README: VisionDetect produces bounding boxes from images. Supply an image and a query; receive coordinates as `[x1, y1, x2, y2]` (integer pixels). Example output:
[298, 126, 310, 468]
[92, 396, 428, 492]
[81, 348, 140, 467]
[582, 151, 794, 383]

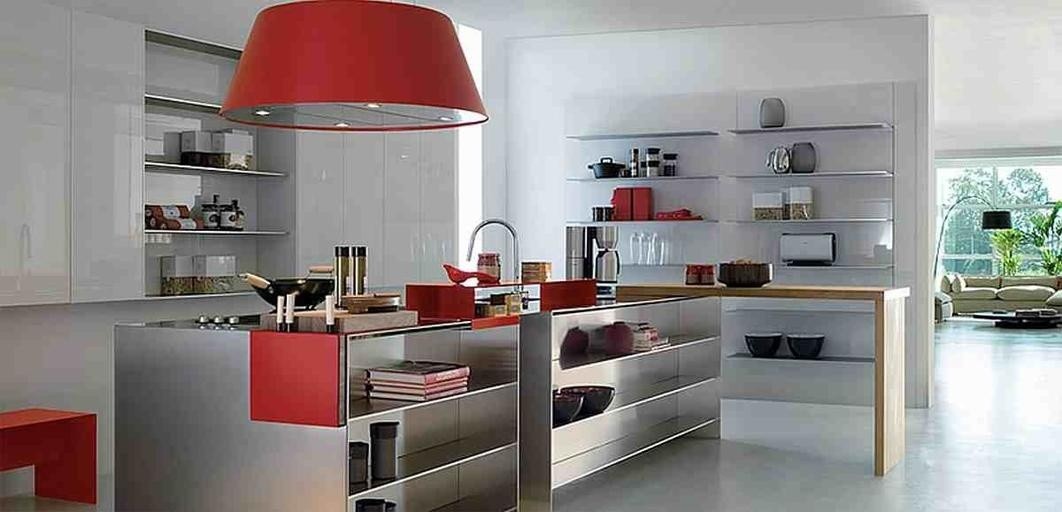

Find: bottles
[370, 422, 400, 478]
[348, 442, 369, 481]
[354, 498, 397, 512]
[630, 148, 678, 177]
[630, 232, 671, 265]
[333, 245, 366, 309]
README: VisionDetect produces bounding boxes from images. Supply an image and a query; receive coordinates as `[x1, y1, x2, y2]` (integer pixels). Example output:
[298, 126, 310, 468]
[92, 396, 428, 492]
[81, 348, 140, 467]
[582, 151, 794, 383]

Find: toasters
[778, 231, 838, 267]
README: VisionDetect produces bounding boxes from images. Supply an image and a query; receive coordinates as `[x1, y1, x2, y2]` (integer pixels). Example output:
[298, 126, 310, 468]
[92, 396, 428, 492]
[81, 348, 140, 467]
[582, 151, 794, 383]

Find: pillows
[952, 274, 966, 293]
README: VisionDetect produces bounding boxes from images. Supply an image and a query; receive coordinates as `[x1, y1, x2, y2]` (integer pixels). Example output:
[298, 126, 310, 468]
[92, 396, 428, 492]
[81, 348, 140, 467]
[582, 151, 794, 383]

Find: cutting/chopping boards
[260, 310, 420, 333]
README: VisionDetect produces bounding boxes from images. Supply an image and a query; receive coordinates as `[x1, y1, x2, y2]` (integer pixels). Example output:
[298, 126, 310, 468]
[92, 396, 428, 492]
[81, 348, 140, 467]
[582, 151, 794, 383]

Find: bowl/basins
[787, 334, 825, 360]
[745, 333, 782, 357]
[561, 386, 615, 413]
[553, 391, 584, 425]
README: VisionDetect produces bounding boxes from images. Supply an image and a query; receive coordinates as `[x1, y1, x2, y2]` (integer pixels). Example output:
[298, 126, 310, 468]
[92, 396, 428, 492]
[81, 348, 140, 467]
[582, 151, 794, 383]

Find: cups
[592, 207, 603, 221]
[605, 207, 616, 220]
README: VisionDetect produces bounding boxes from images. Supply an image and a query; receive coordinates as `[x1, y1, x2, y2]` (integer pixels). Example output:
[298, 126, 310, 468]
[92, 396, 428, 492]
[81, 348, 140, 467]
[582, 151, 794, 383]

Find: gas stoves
[196, 303, 319, 325]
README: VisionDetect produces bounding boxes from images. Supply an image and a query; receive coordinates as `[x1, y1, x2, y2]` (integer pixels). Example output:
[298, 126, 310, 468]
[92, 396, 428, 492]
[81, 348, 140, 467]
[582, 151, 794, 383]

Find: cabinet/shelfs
[258, 126, 459, 298]
[520, 294, 721, 512]
[146, 28, 298, 299]
[0, 0, 146, 308]
[344, 318, 518, 511]
[568, 121, 892, 407]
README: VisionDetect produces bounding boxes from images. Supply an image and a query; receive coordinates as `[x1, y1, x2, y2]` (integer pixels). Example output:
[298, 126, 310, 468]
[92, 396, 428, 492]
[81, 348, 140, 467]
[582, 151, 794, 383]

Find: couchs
[934, 291, 953, 321]
[941, 270, 1062, 322]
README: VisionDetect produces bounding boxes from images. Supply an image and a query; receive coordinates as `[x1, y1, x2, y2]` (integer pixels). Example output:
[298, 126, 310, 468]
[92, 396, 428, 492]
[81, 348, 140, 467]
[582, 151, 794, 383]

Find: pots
[238, 266, 336, 310]
[588, 157, 626, 178]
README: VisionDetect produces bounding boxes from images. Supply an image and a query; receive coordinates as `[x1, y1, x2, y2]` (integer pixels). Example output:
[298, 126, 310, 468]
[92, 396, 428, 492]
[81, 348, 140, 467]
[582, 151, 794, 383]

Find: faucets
[466, 217, 520, 294]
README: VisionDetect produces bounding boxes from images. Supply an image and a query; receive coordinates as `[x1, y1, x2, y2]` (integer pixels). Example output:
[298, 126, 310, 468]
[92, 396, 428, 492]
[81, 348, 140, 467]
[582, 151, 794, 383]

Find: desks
[617, 285, 912, 474]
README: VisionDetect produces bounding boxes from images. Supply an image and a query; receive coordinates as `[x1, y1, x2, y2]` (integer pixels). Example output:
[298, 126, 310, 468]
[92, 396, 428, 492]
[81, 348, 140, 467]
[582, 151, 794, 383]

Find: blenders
[566, 226, 620, 299]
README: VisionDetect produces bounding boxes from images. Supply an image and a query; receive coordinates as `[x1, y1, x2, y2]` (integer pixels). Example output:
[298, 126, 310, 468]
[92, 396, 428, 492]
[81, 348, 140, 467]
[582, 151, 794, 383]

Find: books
[614, 320, 670, 351]
[369, 360, 470, 402]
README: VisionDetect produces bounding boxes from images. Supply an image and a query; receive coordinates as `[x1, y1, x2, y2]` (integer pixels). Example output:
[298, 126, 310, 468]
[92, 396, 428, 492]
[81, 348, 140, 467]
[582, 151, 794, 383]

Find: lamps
[221, 1, 486, 128]
[934, 195, 1012, 276]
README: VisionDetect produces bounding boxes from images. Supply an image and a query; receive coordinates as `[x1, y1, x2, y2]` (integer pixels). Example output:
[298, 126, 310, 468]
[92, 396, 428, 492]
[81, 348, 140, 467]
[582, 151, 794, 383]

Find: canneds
[200, 203, 244, 231]
[522, 262, 552, 283]
[684, 264, 715, 286]
[477, 251, 501, 283]
